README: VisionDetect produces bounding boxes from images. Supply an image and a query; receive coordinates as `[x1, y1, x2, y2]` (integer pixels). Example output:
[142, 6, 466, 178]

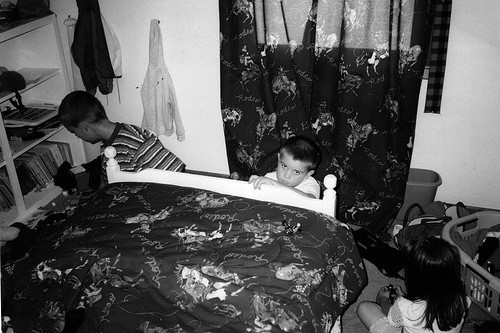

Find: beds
[1, 145, 368, 333]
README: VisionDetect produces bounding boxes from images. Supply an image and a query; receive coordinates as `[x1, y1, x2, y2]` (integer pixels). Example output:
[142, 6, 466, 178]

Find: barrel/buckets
[397, 168, 442, 220]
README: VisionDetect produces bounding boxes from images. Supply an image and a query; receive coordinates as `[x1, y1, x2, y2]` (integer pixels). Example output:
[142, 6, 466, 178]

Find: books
[0, 141, 74, 211]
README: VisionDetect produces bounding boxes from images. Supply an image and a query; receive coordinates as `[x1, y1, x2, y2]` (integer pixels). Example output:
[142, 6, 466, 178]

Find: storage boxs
[440, 210, 500, 321]
[395, 168, 443, 223]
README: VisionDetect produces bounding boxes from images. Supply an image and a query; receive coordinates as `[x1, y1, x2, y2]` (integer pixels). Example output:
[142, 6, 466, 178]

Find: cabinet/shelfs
[0, 12, 88, 228]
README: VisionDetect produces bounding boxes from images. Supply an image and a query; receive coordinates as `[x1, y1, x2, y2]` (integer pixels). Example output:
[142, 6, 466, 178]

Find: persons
[58, 90, 187, 188]
[247, 137, 320, 200]
[357, 235, 471, 333]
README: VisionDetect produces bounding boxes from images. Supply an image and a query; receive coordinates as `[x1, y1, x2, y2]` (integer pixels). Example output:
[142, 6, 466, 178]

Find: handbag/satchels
[393, 203, 453, 252]
